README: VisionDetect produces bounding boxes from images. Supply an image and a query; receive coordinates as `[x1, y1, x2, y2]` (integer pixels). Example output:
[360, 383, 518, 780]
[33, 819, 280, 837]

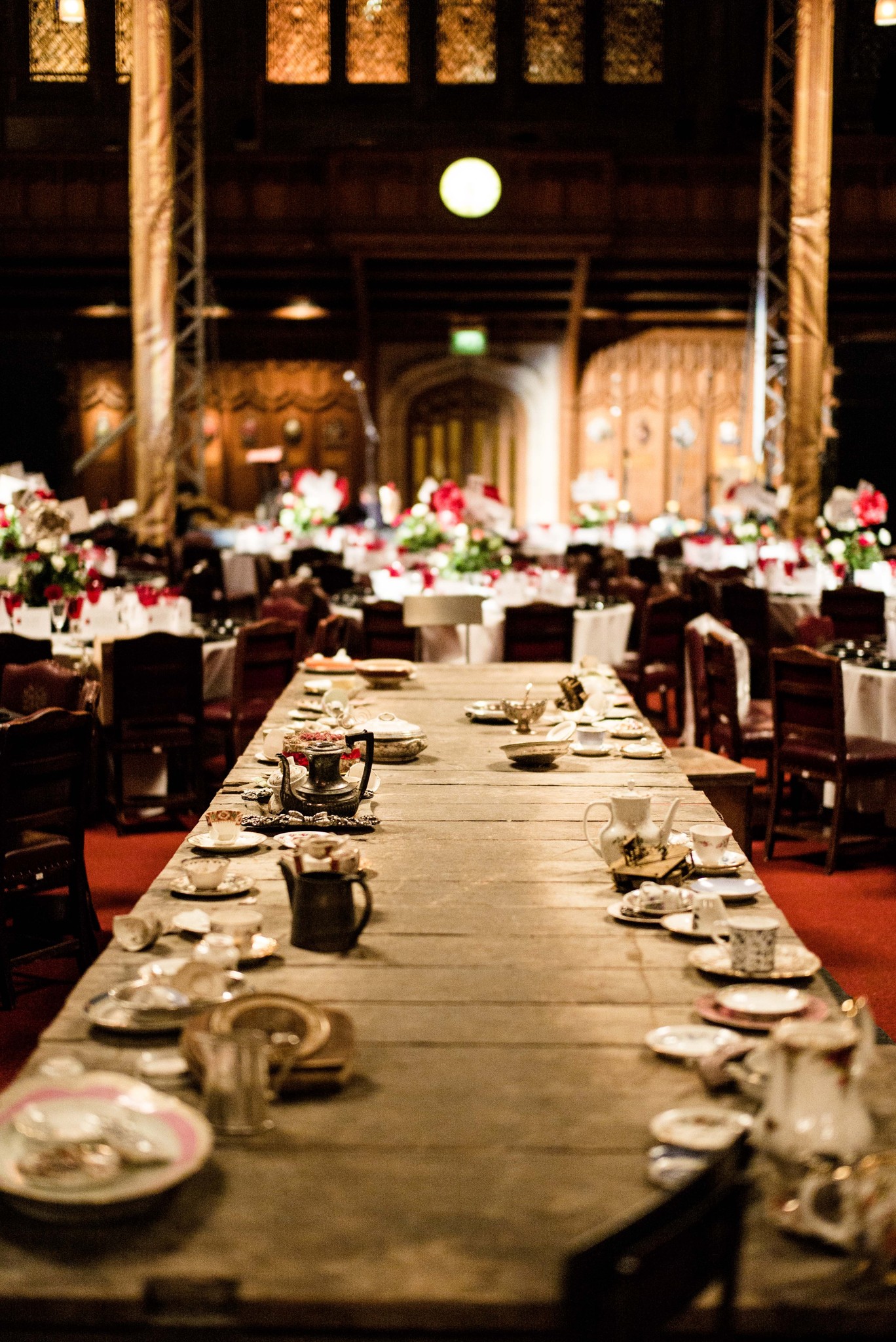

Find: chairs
[0, 507, 894, 998]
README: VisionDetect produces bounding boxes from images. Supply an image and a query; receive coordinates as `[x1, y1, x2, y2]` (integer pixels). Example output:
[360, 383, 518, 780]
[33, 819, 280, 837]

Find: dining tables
[1, 650, 896, 1342]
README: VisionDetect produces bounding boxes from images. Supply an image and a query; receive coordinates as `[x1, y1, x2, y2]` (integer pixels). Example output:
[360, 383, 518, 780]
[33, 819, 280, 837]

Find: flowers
[0, 456, 894, 647]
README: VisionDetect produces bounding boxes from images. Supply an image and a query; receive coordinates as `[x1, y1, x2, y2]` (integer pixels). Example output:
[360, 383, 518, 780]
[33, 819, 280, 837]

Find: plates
[0, 635, 896, 1256]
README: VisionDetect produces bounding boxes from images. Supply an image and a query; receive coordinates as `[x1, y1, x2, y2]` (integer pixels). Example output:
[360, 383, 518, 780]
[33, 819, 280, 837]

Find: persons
[167, 467, 492, 613]
